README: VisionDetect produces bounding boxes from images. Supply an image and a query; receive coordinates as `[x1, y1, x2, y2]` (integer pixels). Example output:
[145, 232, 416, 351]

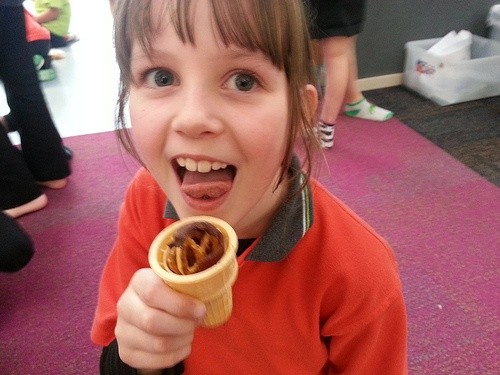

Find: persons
[304, 0, 393, 148]
[0, 0, 72, 217]
[32, 0, 71, 47]
[25, 10, 64, 80]
[88, 0, 409, 375]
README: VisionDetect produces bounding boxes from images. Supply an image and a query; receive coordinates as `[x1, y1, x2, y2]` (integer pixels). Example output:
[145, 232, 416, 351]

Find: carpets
[0, 101, 500, 375]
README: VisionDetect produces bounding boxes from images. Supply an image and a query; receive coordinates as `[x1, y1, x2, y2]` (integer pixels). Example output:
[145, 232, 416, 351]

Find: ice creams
[147, 215, 239, 328]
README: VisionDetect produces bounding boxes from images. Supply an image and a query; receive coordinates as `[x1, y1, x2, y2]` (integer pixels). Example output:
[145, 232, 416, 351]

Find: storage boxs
[403, 36, 500, 106]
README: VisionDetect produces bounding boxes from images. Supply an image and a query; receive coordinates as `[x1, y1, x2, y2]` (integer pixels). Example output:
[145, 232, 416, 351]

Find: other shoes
[38, 178, 67, 188]
[0, 193, 48, 218]
[49, 32, 76, 49]
